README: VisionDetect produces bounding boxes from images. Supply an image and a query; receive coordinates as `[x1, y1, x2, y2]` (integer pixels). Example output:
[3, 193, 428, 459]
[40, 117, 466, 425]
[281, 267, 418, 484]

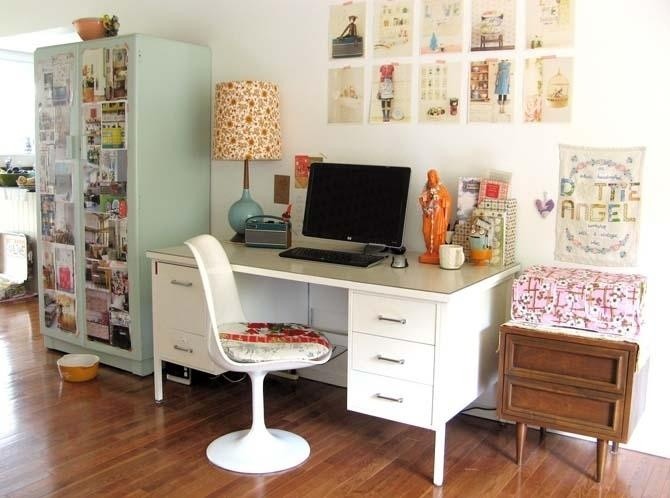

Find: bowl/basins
[0, 173, 28, 187]
[57, 353, 100, 382]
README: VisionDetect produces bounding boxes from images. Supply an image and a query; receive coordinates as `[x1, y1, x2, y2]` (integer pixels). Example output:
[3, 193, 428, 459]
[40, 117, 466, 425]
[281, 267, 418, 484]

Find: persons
[420, 170, 451, 256]
[337, 16, 357, 39]
[377, 63, 394, 121]
[495, 60, 512, 114]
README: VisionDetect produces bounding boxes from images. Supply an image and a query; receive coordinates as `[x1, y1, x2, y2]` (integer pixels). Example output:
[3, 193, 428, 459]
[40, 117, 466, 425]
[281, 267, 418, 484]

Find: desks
[146, 240, 522, 486]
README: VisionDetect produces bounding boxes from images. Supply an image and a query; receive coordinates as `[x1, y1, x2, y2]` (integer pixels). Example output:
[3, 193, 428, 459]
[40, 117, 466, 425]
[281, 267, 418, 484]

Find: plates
[19, 185, 36, 191]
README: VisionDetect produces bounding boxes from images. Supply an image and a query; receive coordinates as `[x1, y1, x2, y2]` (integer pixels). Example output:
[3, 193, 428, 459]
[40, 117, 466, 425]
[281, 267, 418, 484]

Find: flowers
[104, 14, 119, 34]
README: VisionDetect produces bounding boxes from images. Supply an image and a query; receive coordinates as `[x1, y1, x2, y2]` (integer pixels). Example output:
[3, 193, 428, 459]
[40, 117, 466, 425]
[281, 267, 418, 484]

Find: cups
[83, 87, 94, 101]
[439, 244, 465, 269]
[102, 128, 122, 149]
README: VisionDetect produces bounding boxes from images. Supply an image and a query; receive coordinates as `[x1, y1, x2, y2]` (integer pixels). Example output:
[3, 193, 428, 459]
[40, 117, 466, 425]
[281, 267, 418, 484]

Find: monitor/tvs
[302, 162, 411, 255]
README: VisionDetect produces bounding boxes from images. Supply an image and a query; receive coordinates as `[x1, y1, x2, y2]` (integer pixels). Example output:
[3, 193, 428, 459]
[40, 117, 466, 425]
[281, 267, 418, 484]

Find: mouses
[390, 255, 408, 268]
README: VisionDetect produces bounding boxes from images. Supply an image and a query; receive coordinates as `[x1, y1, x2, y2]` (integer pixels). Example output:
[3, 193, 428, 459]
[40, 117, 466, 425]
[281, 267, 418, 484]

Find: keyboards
[279, 247, 384, 269]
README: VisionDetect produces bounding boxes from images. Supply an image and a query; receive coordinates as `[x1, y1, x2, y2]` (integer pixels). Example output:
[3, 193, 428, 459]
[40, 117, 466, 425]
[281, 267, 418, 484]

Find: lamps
[210, 81, 279, 242]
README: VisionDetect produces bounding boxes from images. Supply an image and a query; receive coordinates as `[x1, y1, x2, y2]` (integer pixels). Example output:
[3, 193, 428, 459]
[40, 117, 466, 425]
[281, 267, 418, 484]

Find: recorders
[245, 215, 292, 249]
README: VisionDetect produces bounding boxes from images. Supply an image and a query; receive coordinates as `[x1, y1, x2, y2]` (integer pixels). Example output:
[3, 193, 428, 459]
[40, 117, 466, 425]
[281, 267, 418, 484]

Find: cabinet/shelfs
[33, 33, 213, 376]
[496, 322, 638, 482]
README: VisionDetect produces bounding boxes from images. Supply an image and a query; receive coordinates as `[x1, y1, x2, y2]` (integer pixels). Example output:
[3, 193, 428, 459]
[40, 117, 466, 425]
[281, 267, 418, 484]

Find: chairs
[185, 235, 331, 473]
